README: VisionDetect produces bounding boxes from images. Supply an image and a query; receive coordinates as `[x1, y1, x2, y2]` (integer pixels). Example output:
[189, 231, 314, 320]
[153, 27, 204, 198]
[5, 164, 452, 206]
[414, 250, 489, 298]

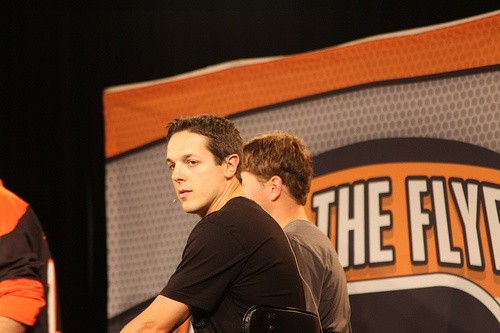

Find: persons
[241, 134, 352, 333]
[119, 114, 306, 333]
[0, 180, 60, 333]
